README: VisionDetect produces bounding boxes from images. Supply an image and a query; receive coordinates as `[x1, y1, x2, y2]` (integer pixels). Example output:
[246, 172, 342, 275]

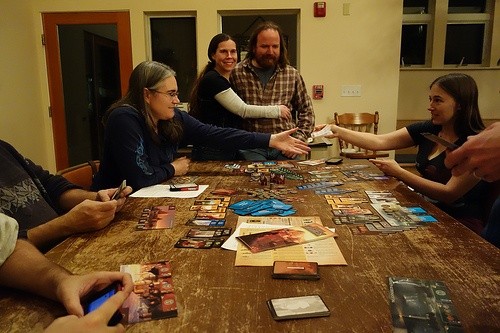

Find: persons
[0, 213, 134, 333]
[92, 61, 312, 197]
[314, 73, 487, 236]
[443, 122, 500, 183]
[0, 139, 133, 254]
[229, 23, 316, 160]
[187, 33, 291, 161]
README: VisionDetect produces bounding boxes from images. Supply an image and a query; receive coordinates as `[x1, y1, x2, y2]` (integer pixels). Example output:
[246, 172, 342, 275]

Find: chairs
[334, 110, 389, 163]
[57, 161, 100, 189]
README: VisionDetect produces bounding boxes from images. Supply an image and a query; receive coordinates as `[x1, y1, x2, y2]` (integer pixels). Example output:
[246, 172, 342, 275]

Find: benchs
[396, 119, 500, 177]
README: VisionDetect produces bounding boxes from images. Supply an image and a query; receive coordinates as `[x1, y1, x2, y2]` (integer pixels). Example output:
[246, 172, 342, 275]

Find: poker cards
[176, 158, 438, 250]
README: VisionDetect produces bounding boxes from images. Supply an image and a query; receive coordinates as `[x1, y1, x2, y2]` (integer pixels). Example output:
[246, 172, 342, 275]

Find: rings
[473, 172, 483, 180]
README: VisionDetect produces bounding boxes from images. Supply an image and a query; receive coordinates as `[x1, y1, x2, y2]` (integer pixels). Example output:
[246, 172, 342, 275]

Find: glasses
[148, 89, 180, 99]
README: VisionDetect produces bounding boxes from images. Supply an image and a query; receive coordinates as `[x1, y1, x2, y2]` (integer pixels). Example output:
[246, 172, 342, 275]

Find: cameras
[80, 281, 127, 326]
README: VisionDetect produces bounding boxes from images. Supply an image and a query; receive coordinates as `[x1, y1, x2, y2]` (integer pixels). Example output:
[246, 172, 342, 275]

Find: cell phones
[325, 157, 343, 165]
[170, 183, 199, 191]
[109, 180, 126, 200]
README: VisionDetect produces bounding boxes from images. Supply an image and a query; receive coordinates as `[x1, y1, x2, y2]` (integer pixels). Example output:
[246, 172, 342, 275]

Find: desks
[0, 159, 500, 333]
